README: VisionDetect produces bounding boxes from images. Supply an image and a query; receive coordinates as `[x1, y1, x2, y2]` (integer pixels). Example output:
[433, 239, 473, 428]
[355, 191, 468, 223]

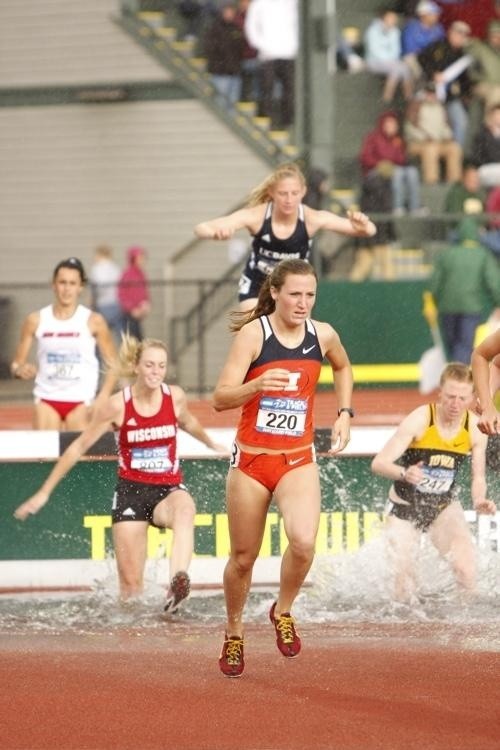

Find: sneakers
[219, 631, 244, 678]
[269, 602, 300, 658]
[164, 572, 189, 614]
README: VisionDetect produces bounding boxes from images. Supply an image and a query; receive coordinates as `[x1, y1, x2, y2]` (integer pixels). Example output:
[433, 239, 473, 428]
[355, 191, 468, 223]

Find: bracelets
[336, 406, 356, 418]
[15, 362, 28, 378]
[401, 468, 410, 483]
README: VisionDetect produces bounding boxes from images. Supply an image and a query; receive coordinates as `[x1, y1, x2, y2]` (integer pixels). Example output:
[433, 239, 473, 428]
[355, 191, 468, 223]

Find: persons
[211, 257, 357, 680]
[434, 215, 499, 366]
[368, 356, 498, 625]
[194, 0, 298, 125]
[471, 331, 500, 436]
[9, 257, 124, 430]
[85, 242, 121, 364]
[12, 339, 230, 623]
[116, 245, 151, 367]
[195, 162, 378, 314]
[338, 1, 499, 253]
